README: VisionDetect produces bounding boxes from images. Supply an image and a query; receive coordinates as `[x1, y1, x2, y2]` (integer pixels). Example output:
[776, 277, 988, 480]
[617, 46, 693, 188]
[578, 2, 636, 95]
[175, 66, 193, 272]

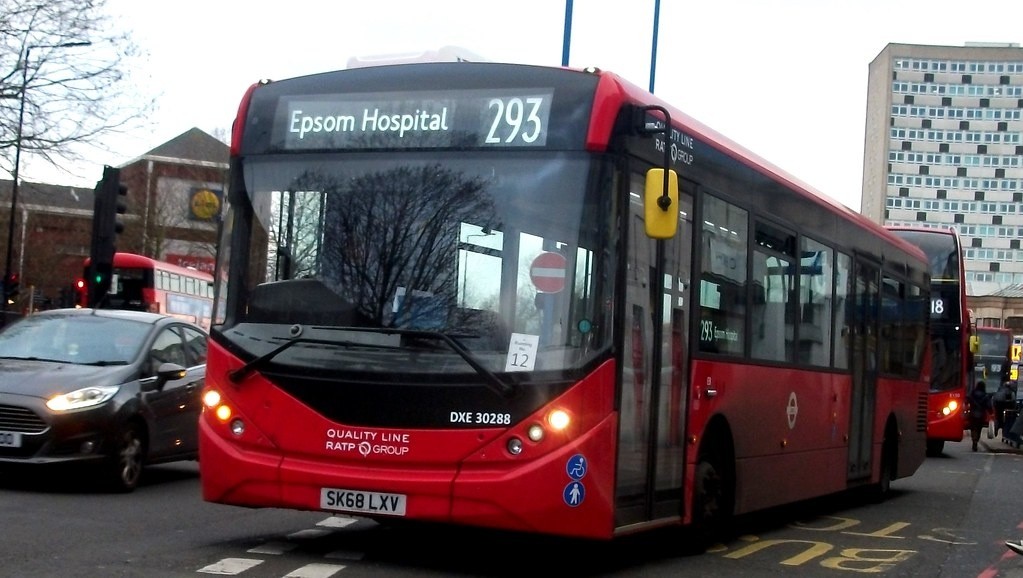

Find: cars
[0, 308, 222, 494]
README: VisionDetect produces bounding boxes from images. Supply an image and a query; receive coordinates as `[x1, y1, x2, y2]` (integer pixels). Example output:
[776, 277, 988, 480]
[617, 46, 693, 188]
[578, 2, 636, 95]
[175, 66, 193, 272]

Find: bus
[198, 56, 932, 550]
[80, 254, 225, 340]
[872, 223, 978, 456]
[970, 325, 1020, 429]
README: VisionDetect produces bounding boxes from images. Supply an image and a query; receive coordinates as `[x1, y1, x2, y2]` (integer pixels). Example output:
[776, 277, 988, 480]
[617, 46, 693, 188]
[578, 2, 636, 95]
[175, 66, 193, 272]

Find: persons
[966, 381, 993, 452]
[992, 380, 1016, 437]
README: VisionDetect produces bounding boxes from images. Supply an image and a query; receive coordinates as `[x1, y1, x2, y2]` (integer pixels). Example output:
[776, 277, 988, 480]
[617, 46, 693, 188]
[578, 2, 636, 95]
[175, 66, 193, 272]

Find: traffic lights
[97, 163, 129, 240]
[4, 273, 20, 299]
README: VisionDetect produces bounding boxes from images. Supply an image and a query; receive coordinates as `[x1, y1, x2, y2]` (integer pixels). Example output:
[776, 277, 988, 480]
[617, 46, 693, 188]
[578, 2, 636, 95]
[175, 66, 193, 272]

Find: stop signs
[530, 251, 569, 294]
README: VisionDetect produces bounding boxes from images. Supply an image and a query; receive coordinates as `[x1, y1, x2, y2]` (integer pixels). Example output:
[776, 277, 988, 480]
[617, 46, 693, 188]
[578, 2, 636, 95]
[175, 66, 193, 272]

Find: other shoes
[972, 447, 977, 452]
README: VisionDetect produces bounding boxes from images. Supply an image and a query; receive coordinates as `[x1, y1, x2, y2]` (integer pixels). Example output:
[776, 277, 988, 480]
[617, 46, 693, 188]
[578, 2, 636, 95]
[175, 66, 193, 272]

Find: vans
[1013, 335, 1023, 365]
